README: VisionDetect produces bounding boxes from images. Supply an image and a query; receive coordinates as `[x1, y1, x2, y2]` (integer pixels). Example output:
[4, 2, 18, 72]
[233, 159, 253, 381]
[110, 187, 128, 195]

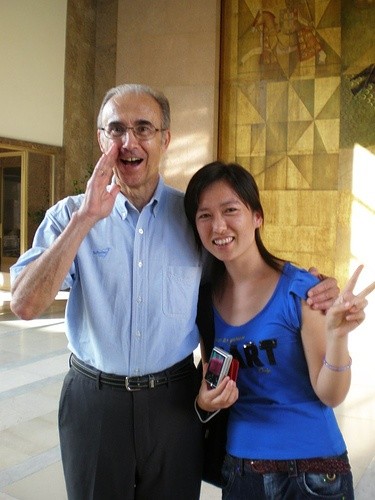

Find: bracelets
[324, 359, 353, 371]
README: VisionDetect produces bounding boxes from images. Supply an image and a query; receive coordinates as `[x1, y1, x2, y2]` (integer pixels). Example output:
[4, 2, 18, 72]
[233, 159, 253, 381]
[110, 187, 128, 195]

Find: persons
[8, 84, 340, 500]
[184, 161, 375, 500]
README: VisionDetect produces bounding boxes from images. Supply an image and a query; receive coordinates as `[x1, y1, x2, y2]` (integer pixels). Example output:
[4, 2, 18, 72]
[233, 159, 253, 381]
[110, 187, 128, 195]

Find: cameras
[204, 346, 233, 389]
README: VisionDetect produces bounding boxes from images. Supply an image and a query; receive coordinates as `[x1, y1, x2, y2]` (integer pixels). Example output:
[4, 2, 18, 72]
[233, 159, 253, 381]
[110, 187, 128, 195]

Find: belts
[233, 458, 352, 476]
[71, 355, 196, 391]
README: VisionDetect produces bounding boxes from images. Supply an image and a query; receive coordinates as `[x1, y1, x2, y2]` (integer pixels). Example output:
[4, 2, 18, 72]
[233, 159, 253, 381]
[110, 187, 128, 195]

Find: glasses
[98, 123, 167, 141]
